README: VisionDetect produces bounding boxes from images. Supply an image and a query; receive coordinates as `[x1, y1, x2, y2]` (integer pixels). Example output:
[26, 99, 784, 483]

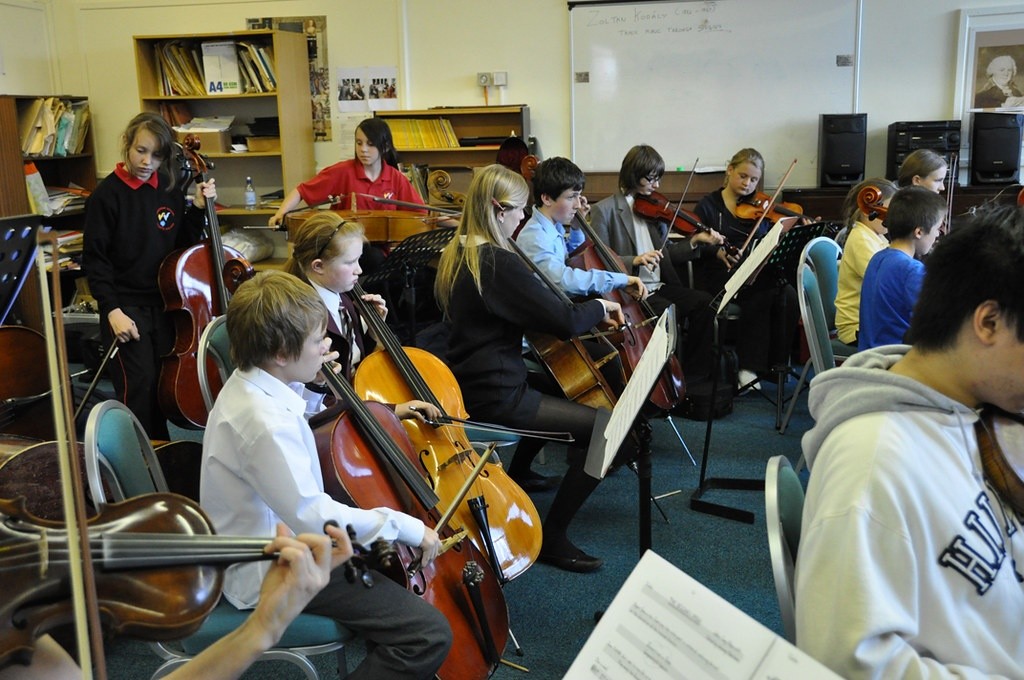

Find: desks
[782, 186, 1023, 231]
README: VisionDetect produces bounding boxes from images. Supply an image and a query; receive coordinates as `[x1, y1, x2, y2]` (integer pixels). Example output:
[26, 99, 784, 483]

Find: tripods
[734, 220, 830, 427]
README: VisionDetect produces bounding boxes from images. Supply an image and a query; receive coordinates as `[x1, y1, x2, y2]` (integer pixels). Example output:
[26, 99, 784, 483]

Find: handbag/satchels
[676, 371, 738, 421]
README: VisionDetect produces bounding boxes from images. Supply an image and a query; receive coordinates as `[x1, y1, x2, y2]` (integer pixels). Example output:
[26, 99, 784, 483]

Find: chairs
[463, 428, 545, 472]
[765, 455, 807, 646]
[197, 315, 235, 415]
[83, 399, 354, 679]
[688, 257, 793, 382]
[779, 227, 858, 435]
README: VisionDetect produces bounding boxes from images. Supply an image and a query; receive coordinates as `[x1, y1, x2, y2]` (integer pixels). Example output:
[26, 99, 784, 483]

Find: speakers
[971, 113, 1023, 187]
[818, 113, 867, 188]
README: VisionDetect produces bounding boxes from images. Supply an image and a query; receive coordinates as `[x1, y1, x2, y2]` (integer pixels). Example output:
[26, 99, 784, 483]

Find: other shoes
[508, 470, 562, 493]
[737, 368, 761, 390]
[537, 537, 604, 571]
[737, 383, 750, 397]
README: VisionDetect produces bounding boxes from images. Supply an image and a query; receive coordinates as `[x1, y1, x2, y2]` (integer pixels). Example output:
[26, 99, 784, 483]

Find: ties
[339, 301, 353, 338]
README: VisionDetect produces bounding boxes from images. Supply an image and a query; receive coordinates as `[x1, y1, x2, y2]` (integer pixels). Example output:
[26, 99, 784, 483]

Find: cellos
[242, 207, 463, 244]
[517, 154, 698, 471]
[856, 185, 952, 260]
[424, 170, 673, 528]
[342, 277, 543, 588]
[154, 132, 259, 432]
[220, 256, 511, 680]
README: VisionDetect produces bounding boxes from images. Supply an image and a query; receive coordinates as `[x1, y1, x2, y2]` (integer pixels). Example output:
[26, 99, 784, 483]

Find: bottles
[244, 177, 256, 210]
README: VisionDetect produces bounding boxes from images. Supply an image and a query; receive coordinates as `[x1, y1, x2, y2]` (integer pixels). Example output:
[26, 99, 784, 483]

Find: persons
[795, 215, 1024, 680]
[857, 184, 949, 353]
[898, 149, 948, 196]
[833, 177, 903, 347]
[0, 111, 823, 680]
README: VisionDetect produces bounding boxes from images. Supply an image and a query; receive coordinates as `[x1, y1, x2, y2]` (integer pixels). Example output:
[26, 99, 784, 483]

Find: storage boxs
[176, 131, 233, 153]
[76, 276, 92, 302]
[201, 41, 245, 95]
[245, 136, 280, 152]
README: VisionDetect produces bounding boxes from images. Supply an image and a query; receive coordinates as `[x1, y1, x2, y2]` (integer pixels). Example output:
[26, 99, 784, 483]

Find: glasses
[644, 175, 659, 184]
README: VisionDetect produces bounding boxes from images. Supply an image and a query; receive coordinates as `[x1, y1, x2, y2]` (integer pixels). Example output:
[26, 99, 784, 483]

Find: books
[153, 39, 277, 96]
[380, 117, 460, 148]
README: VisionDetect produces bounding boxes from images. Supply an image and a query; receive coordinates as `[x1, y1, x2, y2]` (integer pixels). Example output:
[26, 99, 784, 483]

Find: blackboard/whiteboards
[568, 0, 862, 190]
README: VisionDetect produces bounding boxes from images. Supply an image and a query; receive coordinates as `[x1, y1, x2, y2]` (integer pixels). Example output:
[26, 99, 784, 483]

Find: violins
[974, 402, 1024, 526]
[632, 190, 742, 257]
[734, 190, 839, 235]
[0, 488, 401, 673]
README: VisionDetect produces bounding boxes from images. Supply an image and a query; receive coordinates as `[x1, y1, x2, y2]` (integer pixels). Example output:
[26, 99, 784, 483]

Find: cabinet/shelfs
[0, 94, 99, 336]
[132, 29, 316, 271]
[374, 105, 534, 172]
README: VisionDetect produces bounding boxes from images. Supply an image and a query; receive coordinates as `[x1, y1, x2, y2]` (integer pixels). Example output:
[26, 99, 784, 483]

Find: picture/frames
[953, 4, 1024, 167]
